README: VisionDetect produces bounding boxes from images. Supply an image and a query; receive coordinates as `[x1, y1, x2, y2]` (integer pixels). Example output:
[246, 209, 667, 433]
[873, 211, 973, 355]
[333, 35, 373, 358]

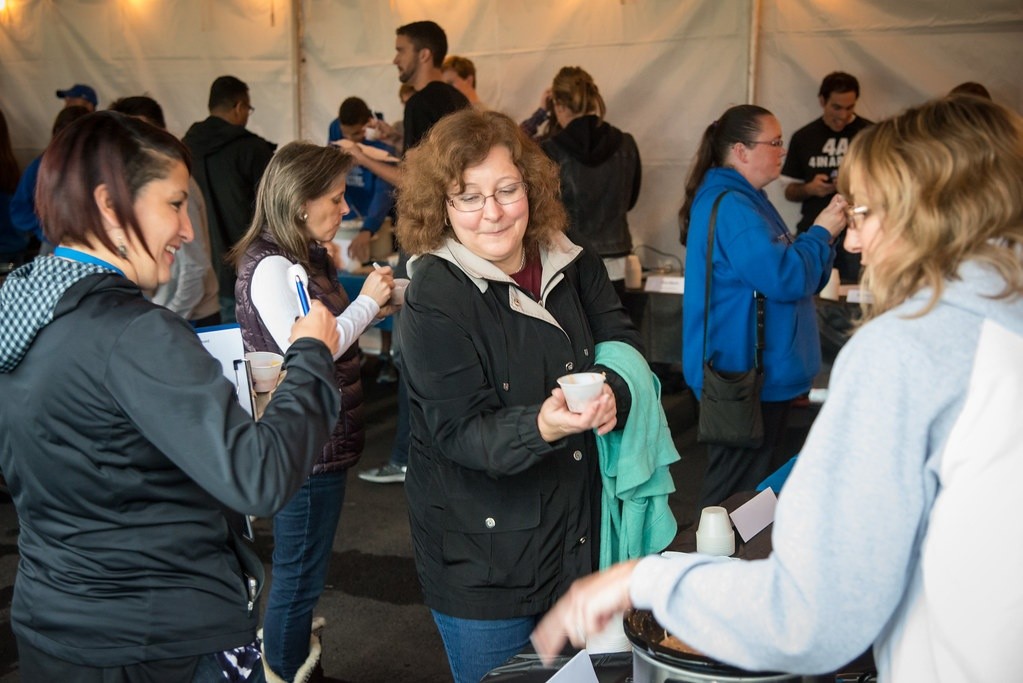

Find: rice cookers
[332, 216, 396, 273]
[622, 606, 837, 682]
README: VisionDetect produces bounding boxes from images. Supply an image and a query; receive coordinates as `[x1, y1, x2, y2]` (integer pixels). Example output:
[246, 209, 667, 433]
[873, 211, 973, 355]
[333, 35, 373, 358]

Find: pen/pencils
[295, 275, 310, 316]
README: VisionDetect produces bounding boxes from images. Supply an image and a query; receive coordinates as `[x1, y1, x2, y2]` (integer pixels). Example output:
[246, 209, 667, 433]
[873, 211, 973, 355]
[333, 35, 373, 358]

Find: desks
[332, 261, 398, 331]
[624, 270, 852, 378]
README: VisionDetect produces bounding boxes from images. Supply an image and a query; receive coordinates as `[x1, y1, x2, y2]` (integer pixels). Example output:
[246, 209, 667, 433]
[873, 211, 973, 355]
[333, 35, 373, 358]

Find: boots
[257, 617, 336, 682]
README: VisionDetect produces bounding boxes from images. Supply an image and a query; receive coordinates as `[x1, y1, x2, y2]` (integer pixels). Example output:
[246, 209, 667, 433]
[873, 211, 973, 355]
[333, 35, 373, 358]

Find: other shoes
[357, 460, 409, 484]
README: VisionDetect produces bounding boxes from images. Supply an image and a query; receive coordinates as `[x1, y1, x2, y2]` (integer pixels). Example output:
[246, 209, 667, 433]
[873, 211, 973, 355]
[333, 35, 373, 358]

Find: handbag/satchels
[696, 360, 767, 449]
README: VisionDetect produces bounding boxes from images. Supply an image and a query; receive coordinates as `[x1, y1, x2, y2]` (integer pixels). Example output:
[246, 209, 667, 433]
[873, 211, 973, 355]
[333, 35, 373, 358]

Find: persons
[677, 104, 849, 510]
[327, 21, 480, 487]
[392, 109, 644, 683]
[522, 68, 640, 309]
[536, 98, 1023, 683]
[0, 109, 343, 683]
[778, 72, 878, 285]
[231, 141, 396, 683]
[0, 75, 278, 338]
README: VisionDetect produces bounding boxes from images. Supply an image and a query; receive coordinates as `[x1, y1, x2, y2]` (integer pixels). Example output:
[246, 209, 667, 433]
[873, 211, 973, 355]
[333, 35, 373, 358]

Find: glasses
[444, 179, 527, 215]
[734, 138, 787, 148]
[841, 204, 877, 229]
[233, 99, 256, 115]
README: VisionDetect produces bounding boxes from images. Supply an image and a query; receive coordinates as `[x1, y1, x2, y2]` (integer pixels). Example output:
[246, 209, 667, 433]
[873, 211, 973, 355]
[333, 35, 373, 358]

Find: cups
[389, 278, 410, 304]
[819, 268, 840, 301]
[625, 254, 641, 288]
[365, 127, 381, 141]
[245, 351, 284, 392]
[696, 506, 735, 557]
[556, 371, 606, 413]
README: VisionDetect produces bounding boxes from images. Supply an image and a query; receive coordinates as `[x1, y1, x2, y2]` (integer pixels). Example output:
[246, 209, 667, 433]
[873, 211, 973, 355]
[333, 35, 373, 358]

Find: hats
[55, 84, 98, 104]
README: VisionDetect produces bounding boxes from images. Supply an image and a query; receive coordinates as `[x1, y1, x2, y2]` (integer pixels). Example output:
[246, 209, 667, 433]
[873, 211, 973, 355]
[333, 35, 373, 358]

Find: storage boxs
[336, 216, 393, 257]
